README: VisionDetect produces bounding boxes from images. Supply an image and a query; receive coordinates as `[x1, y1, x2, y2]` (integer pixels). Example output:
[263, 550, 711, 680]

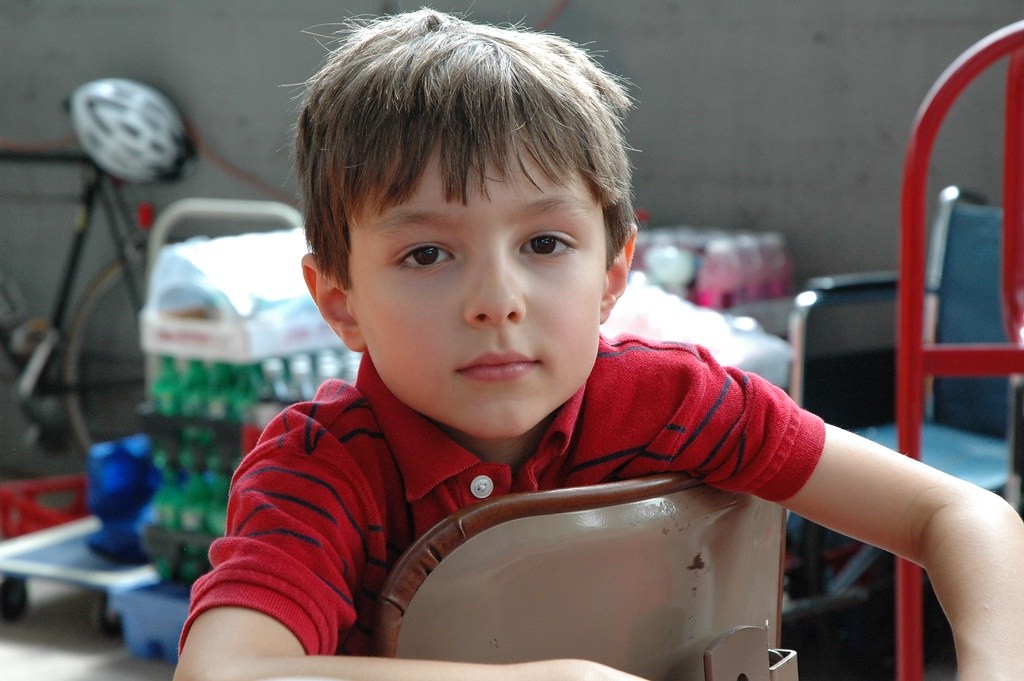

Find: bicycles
[0, 76, 208, 461]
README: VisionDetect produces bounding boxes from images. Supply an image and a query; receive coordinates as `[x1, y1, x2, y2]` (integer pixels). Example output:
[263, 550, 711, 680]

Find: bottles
[636, 224, 792, 310]
[150, 351, 356, 580]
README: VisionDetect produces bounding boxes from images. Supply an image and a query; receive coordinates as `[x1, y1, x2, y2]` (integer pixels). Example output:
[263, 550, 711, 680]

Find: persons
[175, 8, 1024, 681]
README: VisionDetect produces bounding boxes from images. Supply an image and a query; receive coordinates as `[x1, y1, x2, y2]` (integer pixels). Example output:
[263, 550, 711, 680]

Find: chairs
[371, 474, 799, 681]
[825, 186, 1023, 601]
[789, 271, 898, 659]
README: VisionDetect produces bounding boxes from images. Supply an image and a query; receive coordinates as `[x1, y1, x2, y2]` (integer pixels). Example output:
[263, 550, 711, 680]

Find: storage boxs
[137, 308, 341, 363]
[0, 472, 87, 538]
[141, 525, 215, 585]
[133, 399, 244, 473]
